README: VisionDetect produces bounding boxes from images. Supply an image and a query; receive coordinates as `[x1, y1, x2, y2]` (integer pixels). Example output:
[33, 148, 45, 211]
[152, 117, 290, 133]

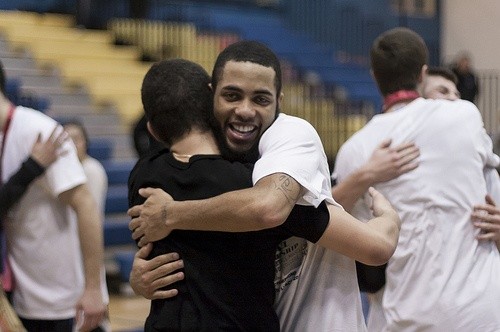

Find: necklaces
[381, 90, 419, 112]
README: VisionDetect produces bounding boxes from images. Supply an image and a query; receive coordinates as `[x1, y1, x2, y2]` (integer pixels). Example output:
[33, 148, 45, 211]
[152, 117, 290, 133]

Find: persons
[59, 117, 108, 221]
[0, 62, 111, 332]
[331, 64, 500, 254]
[126, 59, 402, 332]
[127, 40, 371, 332]
[0, 120, 71, 332]
[332, 26, 500, 332]
